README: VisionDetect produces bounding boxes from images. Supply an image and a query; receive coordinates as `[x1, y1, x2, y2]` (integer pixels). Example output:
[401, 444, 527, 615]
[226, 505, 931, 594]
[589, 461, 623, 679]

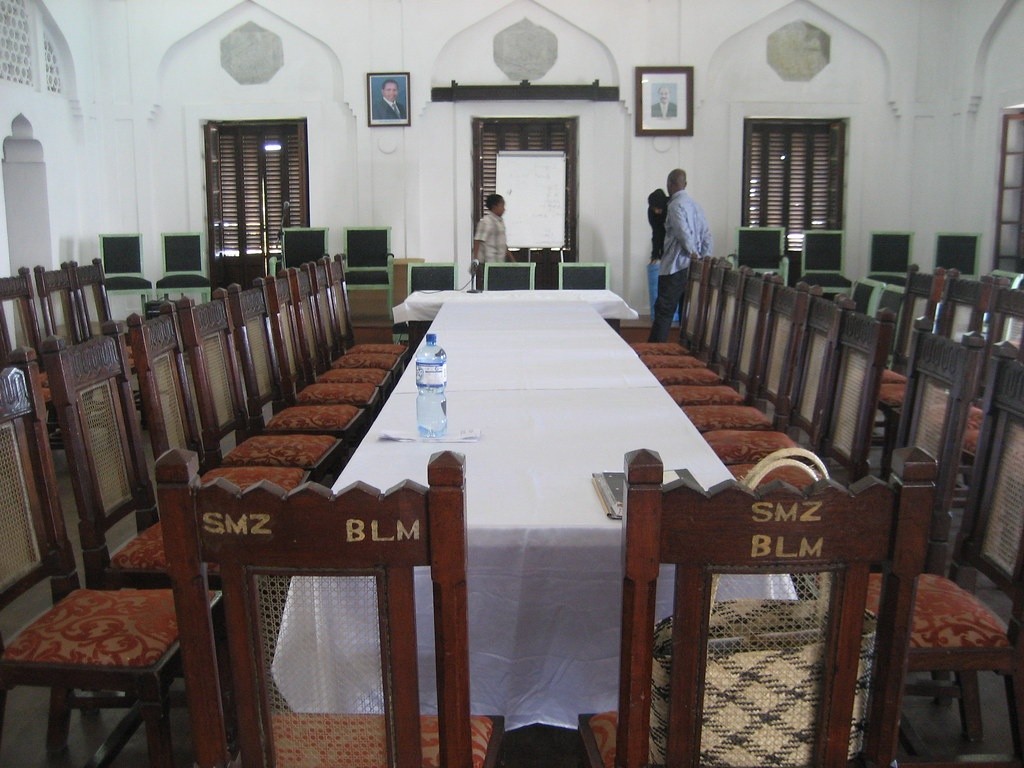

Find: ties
[392, 103, 400, 118]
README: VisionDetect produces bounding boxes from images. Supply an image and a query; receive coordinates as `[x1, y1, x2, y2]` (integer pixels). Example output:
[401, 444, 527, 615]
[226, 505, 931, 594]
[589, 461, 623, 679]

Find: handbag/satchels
[648, 446, 879, 768]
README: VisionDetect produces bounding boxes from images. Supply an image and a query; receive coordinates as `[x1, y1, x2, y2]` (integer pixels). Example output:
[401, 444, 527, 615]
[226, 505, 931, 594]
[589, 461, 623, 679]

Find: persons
[652, 87, 677, 117]
[647, 169, 713, 344]
[373, 80, 406, 119]
[470, 194, 516, 290]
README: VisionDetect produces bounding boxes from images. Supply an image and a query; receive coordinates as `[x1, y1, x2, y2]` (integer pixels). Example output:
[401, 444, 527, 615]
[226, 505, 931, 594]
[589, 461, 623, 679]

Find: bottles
[414, 335, 448, 434]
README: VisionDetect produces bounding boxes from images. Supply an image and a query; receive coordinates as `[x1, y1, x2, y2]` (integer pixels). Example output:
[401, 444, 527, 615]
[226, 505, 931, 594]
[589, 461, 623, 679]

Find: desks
[274, 301, 801, 728]
[392, 289, 640, 355]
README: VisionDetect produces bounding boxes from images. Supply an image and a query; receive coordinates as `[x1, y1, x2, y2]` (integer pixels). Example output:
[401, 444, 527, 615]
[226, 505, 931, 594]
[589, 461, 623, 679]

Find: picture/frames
[635, 66, 694, 138]
[365, 71, 412, 128]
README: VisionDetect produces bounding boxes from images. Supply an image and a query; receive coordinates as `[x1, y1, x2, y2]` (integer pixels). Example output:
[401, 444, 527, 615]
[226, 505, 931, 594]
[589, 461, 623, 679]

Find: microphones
[471, 259, 479, 268]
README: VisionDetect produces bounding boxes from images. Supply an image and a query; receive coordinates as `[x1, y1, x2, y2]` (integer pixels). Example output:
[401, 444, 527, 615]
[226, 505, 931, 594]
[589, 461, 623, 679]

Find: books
[592, 468, 705, 519]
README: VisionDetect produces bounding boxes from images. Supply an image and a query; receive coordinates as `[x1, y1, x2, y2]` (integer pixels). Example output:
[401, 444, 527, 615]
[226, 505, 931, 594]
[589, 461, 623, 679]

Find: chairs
[0, 217, 1024, 768]
